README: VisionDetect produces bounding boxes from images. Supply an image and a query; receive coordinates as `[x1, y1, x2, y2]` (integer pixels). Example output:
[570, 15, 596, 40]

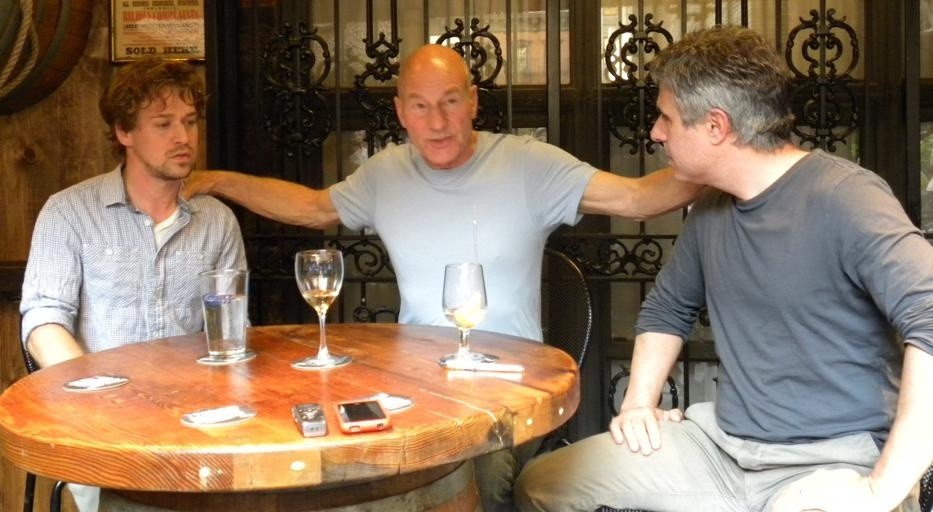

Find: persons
[19, 57, 251, 512]
[183, 40, 705, 512]
[510, 22, 932, 512]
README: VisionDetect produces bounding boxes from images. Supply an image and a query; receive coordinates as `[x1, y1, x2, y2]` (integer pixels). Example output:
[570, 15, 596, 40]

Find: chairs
[536, 246, 592, 452]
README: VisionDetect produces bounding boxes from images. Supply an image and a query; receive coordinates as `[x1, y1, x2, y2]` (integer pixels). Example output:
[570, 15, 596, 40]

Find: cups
[196, 268, 249, 357]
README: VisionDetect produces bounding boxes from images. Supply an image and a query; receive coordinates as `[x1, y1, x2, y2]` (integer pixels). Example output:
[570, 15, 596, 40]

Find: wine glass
[287, 247, 352, 373]
[436, 259, 498, 368]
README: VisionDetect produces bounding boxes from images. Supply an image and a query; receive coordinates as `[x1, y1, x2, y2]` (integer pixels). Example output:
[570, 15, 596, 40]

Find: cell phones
[291, 403, 327, 438]
[334, 400, 391, 434]
